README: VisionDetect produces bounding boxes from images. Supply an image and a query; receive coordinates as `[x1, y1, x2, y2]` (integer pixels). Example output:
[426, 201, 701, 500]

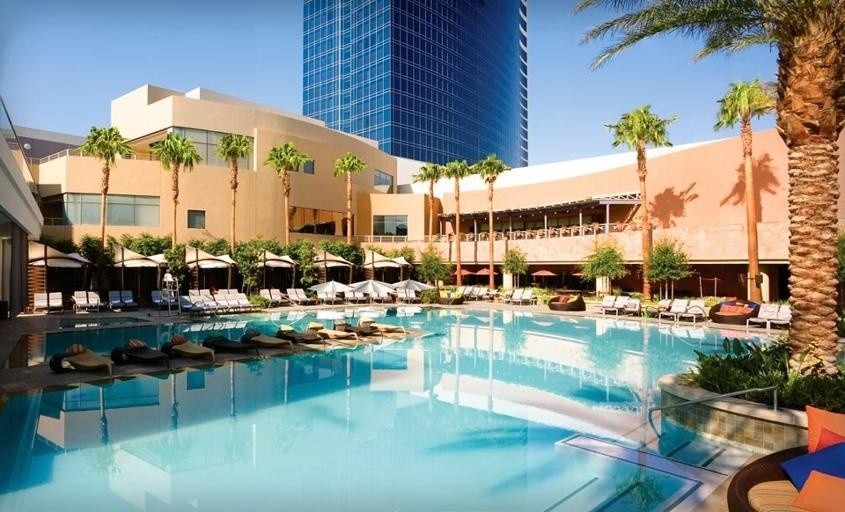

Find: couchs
[434, 292, 465, 305]
[548, 295, 585, 311]
[709, 299, 760, 325]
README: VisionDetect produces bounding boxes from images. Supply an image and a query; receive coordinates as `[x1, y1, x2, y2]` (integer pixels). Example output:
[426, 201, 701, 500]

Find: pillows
[778, 441, 845, 493]
[719, 301, 757, 313]
[815, 428, 845, 453]
[805, 405, 845, 453]
[560, 294, 578, 303]
[440, 291, 463, 298]
[790, 470, 845, 512]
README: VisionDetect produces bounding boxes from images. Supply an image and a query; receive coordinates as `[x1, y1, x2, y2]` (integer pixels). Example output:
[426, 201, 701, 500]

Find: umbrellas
[348, 279, 395, 304]
[572, 270, 584, 276]
[475, 268, 499, 275]
[391, 277, 438, 304]
[531, 270, 557, 284]
[453, 269, 474, 276]
[307, 279, 352, 305]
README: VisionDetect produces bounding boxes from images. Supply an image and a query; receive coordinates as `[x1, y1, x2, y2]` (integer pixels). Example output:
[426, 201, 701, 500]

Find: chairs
[179, 287, 423, 315]
[457, 286, 533, 308]
[726, 444, 807, 512]
[746, 303, 792, 337]
[589, 293, 708, 324]
[31, 288, 175, 314]
